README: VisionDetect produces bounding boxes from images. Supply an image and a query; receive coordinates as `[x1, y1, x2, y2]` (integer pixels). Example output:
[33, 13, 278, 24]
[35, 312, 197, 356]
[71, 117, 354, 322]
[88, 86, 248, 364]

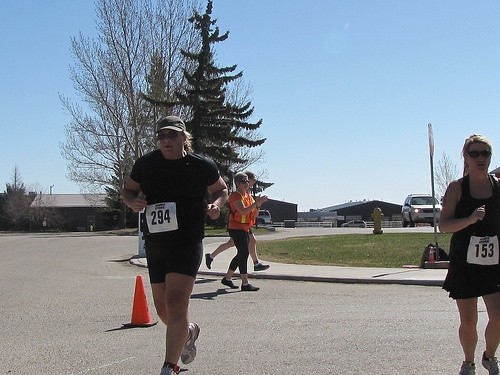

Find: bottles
[428, 246, 435, 263]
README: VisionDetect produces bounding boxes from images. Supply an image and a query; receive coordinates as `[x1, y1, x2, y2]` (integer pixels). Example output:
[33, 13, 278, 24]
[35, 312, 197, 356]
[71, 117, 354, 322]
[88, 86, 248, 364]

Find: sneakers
[482, 351, 500, 375]
[181, 321, 201, 365]
[459, 361, 475, 375]
[160, 364, 176, 375]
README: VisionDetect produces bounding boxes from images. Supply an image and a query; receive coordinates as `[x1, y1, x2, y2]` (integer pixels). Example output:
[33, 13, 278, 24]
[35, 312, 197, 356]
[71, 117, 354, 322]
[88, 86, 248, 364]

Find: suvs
[401, 194, 442, 227]
[255, 209, 272, 226]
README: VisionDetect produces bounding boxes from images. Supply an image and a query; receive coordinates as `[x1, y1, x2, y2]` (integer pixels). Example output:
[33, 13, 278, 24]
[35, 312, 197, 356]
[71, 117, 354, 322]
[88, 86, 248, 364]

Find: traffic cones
[122, 275, 159, 328]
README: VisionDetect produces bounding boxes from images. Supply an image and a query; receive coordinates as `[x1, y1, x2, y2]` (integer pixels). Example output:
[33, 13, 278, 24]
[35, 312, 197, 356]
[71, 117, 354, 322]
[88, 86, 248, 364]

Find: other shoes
[205, 253, 213, 269]
[241, 283, 260, 291]
[221, 278, 239, 289]
[254, 263, 270, 271]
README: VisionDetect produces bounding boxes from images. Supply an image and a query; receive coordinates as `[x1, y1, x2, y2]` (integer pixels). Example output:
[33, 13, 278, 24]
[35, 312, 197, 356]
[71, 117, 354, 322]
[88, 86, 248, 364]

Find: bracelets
[254, 202, 257, 207]
[255, 205, 261, 209]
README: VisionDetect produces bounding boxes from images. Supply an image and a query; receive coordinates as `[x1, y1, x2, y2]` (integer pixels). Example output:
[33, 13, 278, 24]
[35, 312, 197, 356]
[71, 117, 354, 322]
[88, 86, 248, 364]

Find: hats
[156, 115, 186, 133]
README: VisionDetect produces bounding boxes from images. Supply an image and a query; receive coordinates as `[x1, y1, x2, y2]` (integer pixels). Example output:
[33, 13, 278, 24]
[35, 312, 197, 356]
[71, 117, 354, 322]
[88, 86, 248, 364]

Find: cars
[341, 219, 366, 228]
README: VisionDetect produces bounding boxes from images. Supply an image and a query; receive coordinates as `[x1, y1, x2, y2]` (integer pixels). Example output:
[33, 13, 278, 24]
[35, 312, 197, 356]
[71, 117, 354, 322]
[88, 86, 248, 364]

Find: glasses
[158, 131, 183, 140]
[239, 179, 249, 184]
[465, 150, 492, 158]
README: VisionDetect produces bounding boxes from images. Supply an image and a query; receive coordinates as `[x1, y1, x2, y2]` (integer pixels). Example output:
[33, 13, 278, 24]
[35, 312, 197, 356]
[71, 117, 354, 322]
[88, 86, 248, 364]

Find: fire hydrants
[370, 207, 384, 234]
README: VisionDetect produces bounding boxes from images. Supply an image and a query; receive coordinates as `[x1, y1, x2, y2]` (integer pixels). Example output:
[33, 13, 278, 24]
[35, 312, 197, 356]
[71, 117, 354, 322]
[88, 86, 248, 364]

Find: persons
[123, 116, 228, 375]
[222, 172, 268, 292]
[439, 135, 500, 375]
[205, 172, 270, 271]
